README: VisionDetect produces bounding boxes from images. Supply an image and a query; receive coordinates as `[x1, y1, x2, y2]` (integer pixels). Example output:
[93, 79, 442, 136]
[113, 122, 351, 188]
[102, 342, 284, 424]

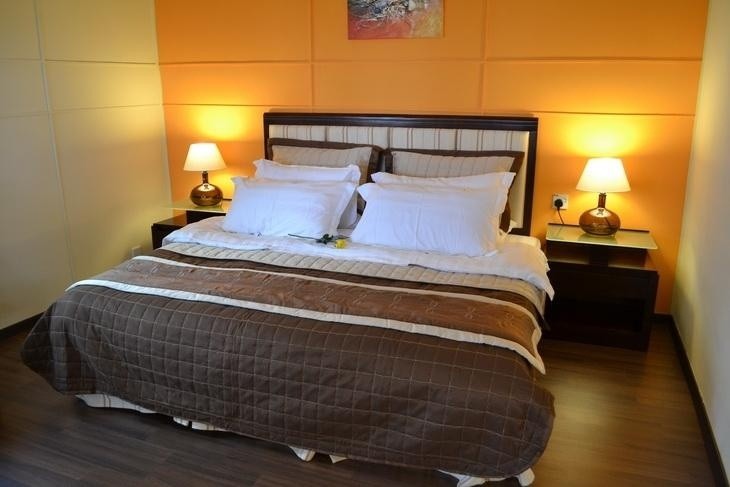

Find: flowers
[287, 233, 349, 249]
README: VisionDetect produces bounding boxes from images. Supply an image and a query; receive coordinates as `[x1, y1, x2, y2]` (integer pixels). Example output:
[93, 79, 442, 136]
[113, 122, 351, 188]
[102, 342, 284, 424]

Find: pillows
[350, 182, 508, 256]
[385, 148, 525, 233]
[370, 171, 517, 190]
[220, 176, 358, 239]
[252, 157, 361, 230]
[265, 136, 384, 217]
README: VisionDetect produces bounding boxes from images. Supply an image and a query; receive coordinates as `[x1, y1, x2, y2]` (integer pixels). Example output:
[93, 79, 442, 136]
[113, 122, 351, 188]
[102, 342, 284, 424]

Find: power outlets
[553, 194, 568, 210]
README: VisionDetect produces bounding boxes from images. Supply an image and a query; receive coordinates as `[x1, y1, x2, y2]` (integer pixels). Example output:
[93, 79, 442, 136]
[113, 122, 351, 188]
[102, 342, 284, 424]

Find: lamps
[183, 143, 227, 207]
[574, 157, 631, 235]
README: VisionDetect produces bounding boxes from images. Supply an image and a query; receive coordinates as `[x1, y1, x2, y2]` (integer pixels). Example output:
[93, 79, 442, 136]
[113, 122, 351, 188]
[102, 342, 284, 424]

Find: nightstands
[150, 199, 231, 250]
[546, 223, 659, 350]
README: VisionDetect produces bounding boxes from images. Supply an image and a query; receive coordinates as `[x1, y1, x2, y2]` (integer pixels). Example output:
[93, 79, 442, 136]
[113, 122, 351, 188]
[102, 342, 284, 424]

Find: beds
[18, 114, 554, 487]
